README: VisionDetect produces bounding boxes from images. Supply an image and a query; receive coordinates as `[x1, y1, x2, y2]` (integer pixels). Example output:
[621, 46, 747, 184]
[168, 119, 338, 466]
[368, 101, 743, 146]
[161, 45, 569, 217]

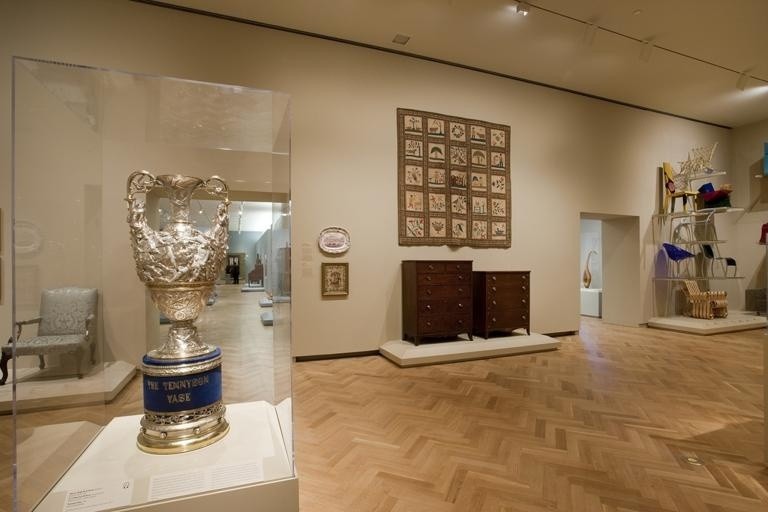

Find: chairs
[663, 141, 737, 318]
[1, 287, 99, 386]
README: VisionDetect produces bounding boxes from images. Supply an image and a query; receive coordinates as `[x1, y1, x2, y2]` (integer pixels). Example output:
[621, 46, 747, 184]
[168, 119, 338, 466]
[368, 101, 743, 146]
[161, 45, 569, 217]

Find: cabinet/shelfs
[472, 271, 530, 338]
[402, 261, 472, 345]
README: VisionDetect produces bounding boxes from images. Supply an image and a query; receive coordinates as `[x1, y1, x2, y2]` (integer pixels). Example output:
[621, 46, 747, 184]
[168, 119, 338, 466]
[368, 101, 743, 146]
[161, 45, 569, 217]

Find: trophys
[122, 168, 232, 456]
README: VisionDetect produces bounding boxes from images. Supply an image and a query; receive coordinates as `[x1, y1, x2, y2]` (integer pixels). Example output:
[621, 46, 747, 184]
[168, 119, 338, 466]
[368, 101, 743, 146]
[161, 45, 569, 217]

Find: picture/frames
[321, 263, 349, 295]
[318, 226, 351, 255]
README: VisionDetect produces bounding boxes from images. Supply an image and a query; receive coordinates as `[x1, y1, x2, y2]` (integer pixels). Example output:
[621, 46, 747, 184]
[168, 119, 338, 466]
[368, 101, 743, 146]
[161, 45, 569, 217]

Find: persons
[229, 262, 240, 285]
